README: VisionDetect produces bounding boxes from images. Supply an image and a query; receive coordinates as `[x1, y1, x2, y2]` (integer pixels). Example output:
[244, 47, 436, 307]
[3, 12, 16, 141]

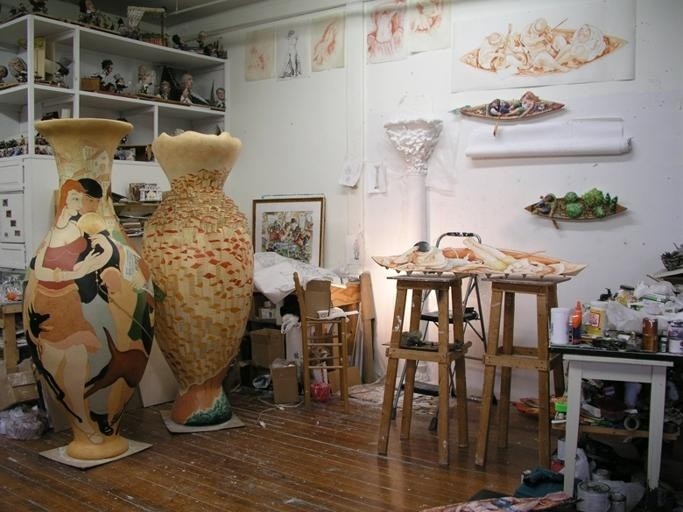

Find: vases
[24, 120, 155, 459]
[143, 132, 252, 425]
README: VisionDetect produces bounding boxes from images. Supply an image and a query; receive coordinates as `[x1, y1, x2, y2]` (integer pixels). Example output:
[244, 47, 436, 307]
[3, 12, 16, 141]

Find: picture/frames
[252, 197, 326, 269]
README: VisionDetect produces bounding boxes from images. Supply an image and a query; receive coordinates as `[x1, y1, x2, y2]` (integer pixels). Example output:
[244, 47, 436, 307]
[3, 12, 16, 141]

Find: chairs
[392, 233, 498, 430]
[293, 272, 349, 415]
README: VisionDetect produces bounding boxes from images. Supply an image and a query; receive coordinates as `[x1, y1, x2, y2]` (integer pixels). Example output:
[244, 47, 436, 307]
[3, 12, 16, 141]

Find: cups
[551, 308, 570, 344]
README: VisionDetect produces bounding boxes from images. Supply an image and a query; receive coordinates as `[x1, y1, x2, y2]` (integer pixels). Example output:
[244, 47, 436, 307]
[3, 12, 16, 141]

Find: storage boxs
[260, 308, 277, 320]
[327, 367, 362, 393]
[0, 371, 39, 410]
[272, 359, 299, 403]
[305, 279, 331, 320]
[251, 328, 284, 368]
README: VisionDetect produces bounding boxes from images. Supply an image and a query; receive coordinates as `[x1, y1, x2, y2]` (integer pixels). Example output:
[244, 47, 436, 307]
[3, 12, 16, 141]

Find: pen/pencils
[646, 274, 660, 282]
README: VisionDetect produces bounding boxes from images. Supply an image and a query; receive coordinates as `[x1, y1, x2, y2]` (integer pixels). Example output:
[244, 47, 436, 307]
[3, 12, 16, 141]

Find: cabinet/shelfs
[0, 303, 28, 374]
[0, 15, 230, 269]
[228, 281, 364, 400]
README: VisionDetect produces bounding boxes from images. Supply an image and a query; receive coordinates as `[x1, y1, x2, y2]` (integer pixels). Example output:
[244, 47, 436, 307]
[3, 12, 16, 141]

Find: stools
[563, 354, 673, 497]
[391, 276, 472, 436]
[475, 276, 566, 467]
